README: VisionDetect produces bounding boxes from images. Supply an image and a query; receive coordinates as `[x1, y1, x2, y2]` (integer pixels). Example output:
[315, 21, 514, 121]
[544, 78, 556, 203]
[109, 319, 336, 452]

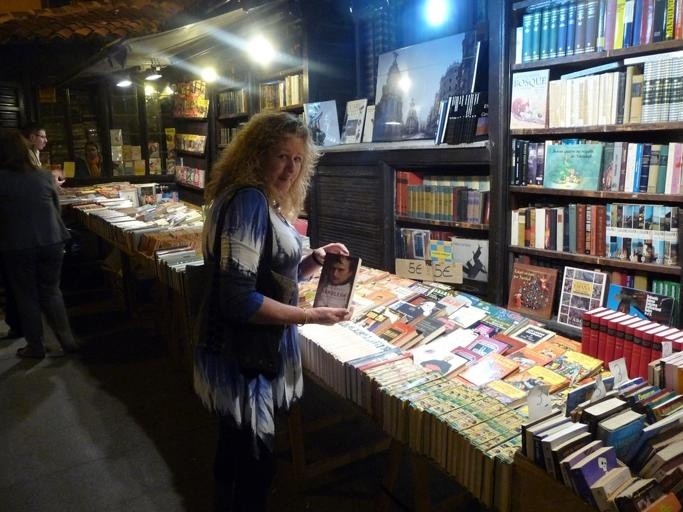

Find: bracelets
[296, 306, 307, 328]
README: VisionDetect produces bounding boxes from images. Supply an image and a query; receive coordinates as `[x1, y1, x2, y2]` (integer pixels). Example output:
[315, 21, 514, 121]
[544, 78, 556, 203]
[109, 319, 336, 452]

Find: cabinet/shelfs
[384, 152, 496, 302]
[204, 19, 354, 220]
[157, 53, 204, 202]
[496, 0, 683, 365]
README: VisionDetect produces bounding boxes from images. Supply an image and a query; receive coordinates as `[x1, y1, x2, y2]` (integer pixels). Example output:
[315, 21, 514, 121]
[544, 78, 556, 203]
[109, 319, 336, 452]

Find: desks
[53, 177, 683, 511]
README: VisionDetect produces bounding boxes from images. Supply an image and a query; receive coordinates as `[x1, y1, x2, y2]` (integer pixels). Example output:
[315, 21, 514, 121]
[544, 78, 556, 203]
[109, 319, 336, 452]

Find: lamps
[143, 59, 162, 81]
[114, 71, 133, 89]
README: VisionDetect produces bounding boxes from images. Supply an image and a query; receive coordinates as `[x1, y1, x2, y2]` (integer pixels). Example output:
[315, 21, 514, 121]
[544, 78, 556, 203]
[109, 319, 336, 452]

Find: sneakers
[16, 345, 46, 360]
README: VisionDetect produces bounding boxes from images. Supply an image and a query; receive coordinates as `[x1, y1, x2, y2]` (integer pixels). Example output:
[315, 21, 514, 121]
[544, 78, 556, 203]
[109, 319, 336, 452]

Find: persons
[187, 106, 354, 509]
[2, 123, 77, 357]
[315, 256, 352, 310]
[78, 141, 104, 176]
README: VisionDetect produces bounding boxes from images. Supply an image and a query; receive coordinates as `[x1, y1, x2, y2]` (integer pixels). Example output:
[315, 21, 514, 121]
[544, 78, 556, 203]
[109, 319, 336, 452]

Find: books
[147, 143, 161, 175]
[220, 124, 249, 151]
[175, 165, 206, 189]
[165, 129, 176, 176]
[342, 99, 376, 147]
[217, 90, 247, 118]
[510, 52, 682, 131]
[511, 203, 681, 266]
[58, 181, 204, 294]
[435, 93, 488, 146]
[509, 138, 681, 196]
[392, 227, 488, 282]
[110, 126, 145, 176]
[259, 75, 300, 111]
[508, 2, 682, 65]
[395, 171, 490, 222]
[507, 258, 682, 338]
[290, 255, 613, 510]
[175, 133, 207, 152]
[520, 306, 681, 511]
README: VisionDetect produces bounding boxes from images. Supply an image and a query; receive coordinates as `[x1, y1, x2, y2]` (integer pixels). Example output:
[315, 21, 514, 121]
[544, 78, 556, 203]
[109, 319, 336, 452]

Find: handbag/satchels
[181, 185, 300, 361]
